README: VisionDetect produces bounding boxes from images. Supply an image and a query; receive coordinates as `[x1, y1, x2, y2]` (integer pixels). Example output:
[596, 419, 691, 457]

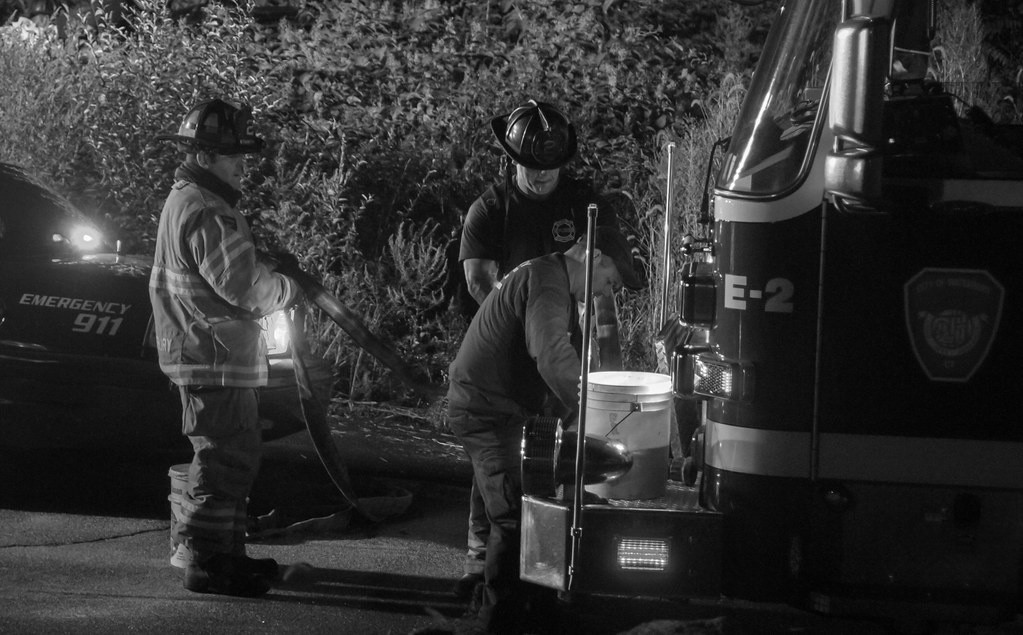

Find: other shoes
[452, 564, 485, 596]
[176, 544, 281, 595]
[476, 605, 539, 635]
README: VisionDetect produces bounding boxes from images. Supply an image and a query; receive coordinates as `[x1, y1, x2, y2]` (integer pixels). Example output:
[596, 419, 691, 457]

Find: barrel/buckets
[578, 371, 672, 502]
[168, 463, 192, 568]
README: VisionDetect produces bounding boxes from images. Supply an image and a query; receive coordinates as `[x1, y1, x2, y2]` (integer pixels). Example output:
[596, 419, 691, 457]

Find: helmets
[153, 98, 260, 154]
[490, 98, 578, 168]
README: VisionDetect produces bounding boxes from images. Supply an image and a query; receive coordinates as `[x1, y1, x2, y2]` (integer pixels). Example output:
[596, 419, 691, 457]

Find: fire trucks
[0, 163, 330, 444]
[521, 0, 1023, 635]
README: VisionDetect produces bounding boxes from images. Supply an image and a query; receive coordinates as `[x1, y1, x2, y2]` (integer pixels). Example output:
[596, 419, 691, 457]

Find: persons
[458, 98, 619, 560]
[446, 227, 650, 635]
[148, 100, 308, 598]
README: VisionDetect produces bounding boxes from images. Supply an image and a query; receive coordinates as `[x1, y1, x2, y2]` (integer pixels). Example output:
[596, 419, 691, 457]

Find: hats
[577, 218, 651, 290]
